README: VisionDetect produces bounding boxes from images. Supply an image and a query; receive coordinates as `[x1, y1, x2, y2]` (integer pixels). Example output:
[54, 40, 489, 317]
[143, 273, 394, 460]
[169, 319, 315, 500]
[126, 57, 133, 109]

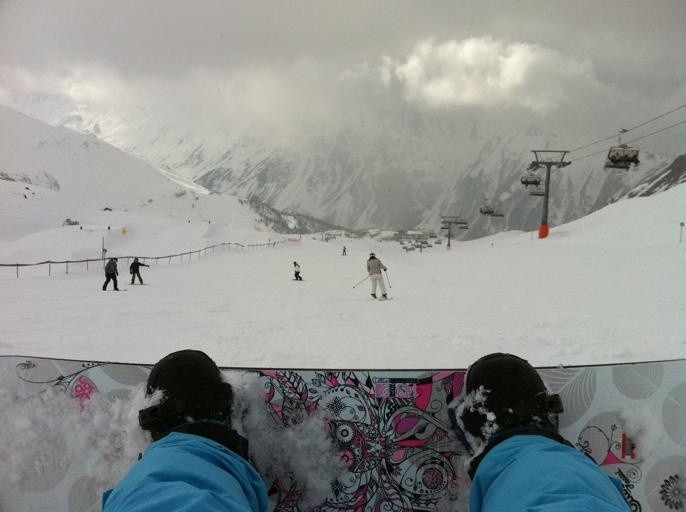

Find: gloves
[384, 269, 387, 271]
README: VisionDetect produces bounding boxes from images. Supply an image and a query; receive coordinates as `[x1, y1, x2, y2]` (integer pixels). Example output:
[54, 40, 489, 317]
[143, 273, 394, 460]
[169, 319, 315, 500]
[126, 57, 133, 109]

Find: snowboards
[2, 356, 686, 512]
[103, 289, 127, 291]
[128, 283, 146, 286]
[293, 278, 304, 281]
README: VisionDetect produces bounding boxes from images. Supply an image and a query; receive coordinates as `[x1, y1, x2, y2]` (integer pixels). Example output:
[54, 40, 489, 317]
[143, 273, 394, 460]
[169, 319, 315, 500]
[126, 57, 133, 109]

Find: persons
[366, 253, 387, 298]
[293, 261, 302, 280]
[102, 350, 632, 512]
[611, 150, 641, 168]
[129, 258, 149, 285]
[343, 246, 346, 255]
[102, 257, 119, 290]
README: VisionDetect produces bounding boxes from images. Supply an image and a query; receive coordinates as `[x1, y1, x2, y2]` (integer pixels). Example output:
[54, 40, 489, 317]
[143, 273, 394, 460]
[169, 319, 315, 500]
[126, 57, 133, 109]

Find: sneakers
[464, 352, 565, 467]
[131, 282, 143, 284]
[296, 278, 302, 280]
[138, 348, 237, 450]
[103, 288, 106, 290]
[372, 295, 387, 298]
[114, 288, 118, 290]
